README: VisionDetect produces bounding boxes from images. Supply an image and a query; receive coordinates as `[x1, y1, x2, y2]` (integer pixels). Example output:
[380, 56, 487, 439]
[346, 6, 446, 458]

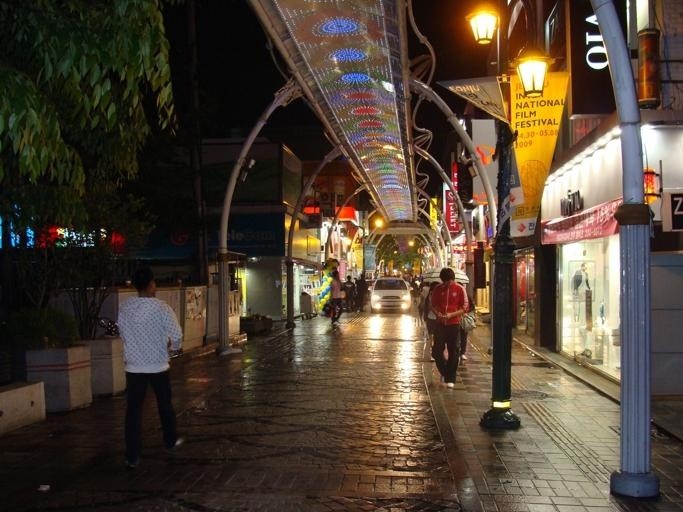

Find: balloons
[307, 257, 339, 316]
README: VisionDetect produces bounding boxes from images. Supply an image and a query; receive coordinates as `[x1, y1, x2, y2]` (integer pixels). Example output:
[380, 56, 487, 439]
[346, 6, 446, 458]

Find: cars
[366, 275, 414, 316]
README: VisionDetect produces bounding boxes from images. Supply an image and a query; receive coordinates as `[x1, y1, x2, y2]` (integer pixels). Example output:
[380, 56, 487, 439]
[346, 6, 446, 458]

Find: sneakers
[439, 375, 455, 388]
[460, 355, 467, 360]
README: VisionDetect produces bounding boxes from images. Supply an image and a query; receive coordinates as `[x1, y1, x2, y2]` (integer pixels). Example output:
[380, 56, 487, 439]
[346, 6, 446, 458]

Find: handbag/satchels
[463, 315, 476, 330]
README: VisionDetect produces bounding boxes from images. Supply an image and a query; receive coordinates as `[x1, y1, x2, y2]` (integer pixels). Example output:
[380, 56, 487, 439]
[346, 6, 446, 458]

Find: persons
[456, 281, 478, 361]
[116, 267, 188, 468]
[329, 272, 367, 326]
[429, 266, 470, 389]
[424, 281, 441, 361]
[574, 259, 593, 300]
[408, 280, 430, 325]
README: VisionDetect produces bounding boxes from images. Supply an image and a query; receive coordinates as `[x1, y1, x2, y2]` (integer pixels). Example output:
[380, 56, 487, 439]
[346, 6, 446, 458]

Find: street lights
[461, 0, 560, 432]
[350, 214, 383, 313]
